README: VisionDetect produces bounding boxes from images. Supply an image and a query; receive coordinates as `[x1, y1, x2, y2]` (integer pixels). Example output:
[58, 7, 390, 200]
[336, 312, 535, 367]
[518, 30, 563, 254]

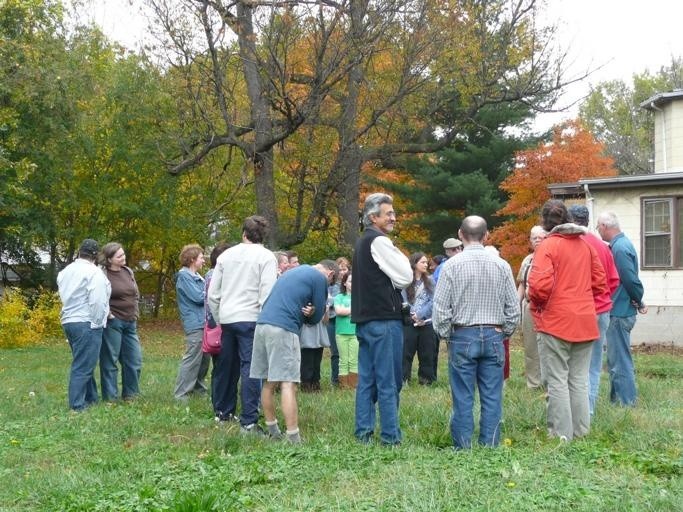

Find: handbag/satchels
[200, 277, 223, 354]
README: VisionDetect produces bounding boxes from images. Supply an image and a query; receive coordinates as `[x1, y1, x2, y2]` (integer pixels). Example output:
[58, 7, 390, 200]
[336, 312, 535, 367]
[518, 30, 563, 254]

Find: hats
[566, 205, 590, 225]
[78, 238, 99, 255]
[443, 237, 461, 248]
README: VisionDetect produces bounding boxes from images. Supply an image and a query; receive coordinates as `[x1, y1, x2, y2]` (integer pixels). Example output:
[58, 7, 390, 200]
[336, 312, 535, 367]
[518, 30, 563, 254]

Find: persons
[433, 238, 463, 286]
[525, 201, 608, 439]
[568, 205, 620, 420]
[597, 212, 648, 407]
[350, 193, 413, 447]
[101, 242, 141, 402]
[429, 254, 445, 274]
[326, 257, 351, 388]
[274, 252, 289, 276]
[432, 215, 521, 449]
[176, 243, 210, 398]
[334, 271, 358, 389]
[401, 252, 439, 388]
[515, 226, 549, 389]
[56, 239, 111, 409]
[287, 253, 299, 269]
[207, 215, 278, 435]
[482, 245, 511, 381]
[203, 244, 231, 412]
[249, 260, 339, 443]
[299, 320, 331, 393]
